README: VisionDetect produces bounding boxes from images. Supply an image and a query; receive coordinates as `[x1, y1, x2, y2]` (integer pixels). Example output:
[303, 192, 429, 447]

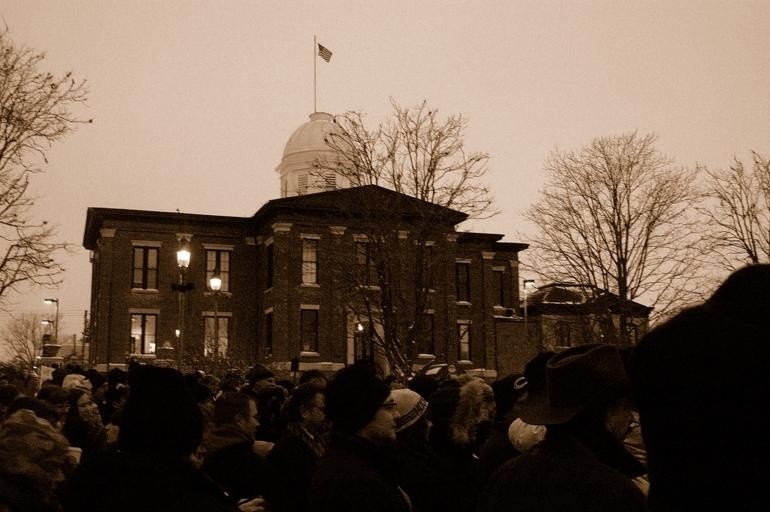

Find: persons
[0, 264, 770, 511]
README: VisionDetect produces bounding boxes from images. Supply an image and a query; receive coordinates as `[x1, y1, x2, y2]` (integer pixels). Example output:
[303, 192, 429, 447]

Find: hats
[390, 388, 433, 434]
[515, 340, 633, 426]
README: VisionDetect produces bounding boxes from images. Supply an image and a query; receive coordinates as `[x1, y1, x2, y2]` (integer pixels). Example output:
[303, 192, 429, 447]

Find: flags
[316, 42, 333, 64]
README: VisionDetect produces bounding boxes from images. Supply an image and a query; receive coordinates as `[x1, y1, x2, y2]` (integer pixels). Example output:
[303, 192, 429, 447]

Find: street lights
[355, 323, 364, 360]
[175, 233, 193, 370]
[32, 299, 59, 344]
[523, 278, 536, 320]
[204, 268, 232, 360]
